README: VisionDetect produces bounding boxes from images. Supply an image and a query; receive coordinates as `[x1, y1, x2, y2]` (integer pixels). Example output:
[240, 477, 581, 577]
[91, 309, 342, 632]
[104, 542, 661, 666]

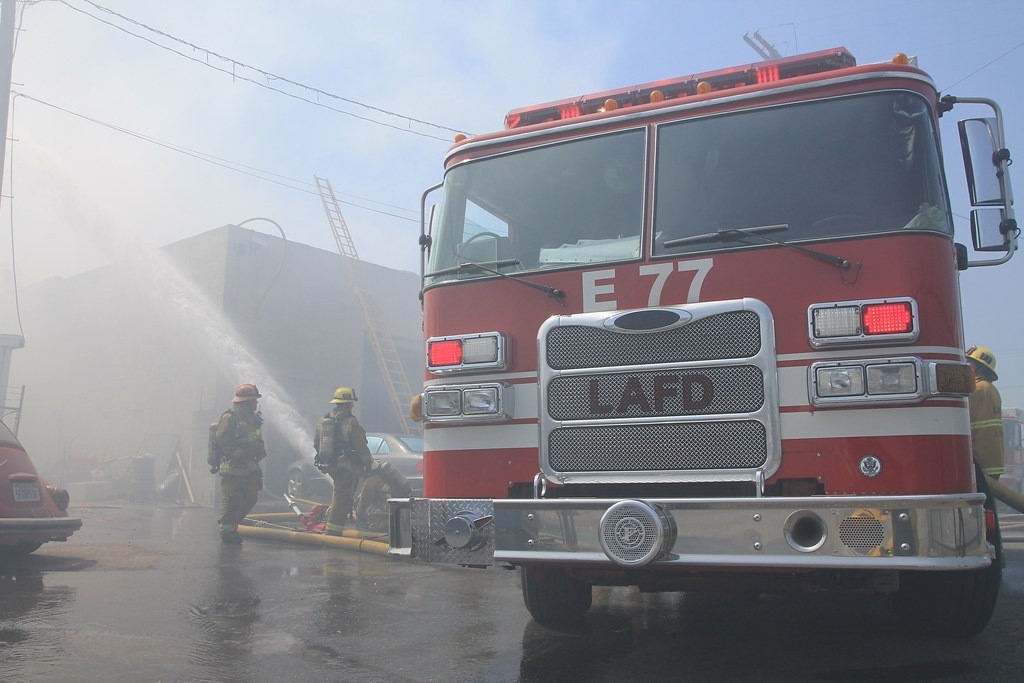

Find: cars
[286, 431, 425, 510]
[1, 420, 84, 560]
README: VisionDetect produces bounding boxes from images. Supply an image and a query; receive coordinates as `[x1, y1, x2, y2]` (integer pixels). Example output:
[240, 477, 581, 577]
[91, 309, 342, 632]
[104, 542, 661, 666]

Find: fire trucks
[418, 44, 1024, 640]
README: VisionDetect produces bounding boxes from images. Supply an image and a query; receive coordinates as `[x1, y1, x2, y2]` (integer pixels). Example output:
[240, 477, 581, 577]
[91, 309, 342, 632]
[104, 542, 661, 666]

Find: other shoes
[220, 531, 243, 544]
[355, 505, 367, 516]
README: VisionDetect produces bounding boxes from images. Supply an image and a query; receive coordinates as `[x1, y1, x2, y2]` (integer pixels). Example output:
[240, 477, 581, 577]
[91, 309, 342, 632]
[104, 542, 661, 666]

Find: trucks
[997, 407, 1023, 493]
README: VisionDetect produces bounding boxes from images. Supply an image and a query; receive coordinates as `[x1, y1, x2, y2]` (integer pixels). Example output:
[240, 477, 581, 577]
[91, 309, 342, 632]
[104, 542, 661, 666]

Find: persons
[315, 387, 379, 536]
[965, 344, 1006, 569]
[216, 384, 266, 543]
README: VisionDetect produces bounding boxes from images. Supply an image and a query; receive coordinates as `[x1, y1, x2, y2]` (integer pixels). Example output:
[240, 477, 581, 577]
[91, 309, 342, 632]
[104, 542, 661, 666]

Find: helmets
[231, 384, 261, 403]
[967, 346, 998, 380]
[329, 386, 357, 403]
[363, 459, 379, 471]
